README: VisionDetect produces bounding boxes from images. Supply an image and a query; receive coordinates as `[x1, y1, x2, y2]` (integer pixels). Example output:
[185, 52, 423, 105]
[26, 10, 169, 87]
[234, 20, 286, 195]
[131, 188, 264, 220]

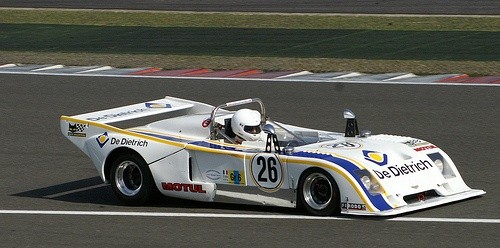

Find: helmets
[231, 108, 263, 142]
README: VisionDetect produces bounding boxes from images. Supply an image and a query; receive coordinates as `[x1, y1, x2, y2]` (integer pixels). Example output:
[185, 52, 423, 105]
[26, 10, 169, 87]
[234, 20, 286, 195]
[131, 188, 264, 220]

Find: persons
[231, 108, 300, 148]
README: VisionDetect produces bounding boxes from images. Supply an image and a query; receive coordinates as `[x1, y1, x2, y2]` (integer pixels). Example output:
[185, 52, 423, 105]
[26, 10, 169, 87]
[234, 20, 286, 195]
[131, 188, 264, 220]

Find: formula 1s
[60, 96, 486, 217]
